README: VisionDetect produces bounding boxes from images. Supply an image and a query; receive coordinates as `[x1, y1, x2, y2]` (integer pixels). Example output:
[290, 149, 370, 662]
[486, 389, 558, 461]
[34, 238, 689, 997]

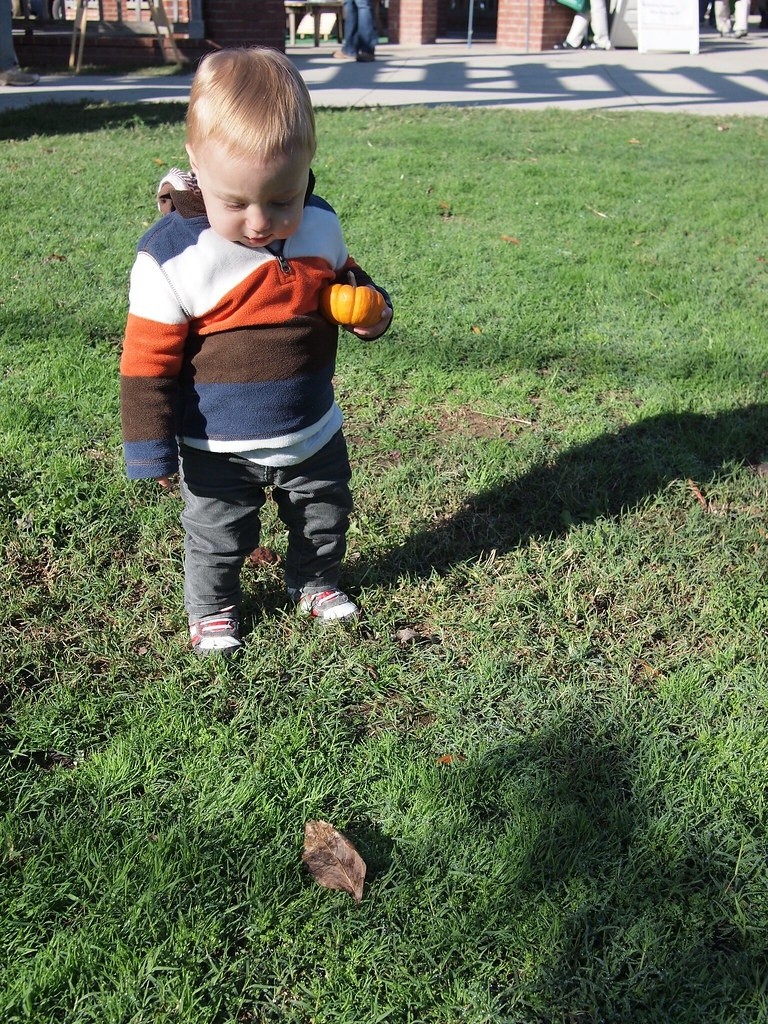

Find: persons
[0, 0, 40, 87]
[553, 0, 613, 50]
[699, 0, 768, 38]
[121, 46, 393, 651]
[332, 0, 377, 62]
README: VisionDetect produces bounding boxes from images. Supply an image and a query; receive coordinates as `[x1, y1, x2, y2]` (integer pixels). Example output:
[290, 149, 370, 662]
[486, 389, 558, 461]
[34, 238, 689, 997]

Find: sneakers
[190, 604, 241, 656]
[287, 587, 359, 622]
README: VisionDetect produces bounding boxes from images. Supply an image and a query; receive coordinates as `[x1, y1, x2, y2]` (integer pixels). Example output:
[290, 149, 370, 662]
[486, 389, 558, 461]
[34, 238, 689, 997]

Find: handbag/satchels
[557, 0, 588, 13]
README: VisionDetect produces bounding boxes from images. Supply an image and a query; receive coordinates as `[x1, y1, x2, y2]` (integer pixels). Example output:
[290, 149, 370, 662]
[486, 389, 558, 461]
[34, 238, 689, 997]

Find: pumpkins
[319, 270, 385, 328]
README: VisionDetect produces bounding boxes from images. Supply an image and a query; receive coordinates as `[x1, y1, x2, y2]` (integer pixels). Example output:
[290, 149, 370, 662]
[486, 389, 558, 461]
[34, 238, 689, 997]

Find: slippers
[582, 43, 606, 50]
[552, 41, 579, 49]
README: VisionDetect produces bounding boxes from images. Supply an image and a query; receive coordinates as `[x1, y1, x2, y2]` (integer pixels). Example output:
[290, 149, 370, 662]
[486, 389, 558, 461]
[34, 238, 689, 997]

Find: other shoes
[735, 31, 748, 38]
[357, 50, 375, 62]
[332, 50, 357, 59]
